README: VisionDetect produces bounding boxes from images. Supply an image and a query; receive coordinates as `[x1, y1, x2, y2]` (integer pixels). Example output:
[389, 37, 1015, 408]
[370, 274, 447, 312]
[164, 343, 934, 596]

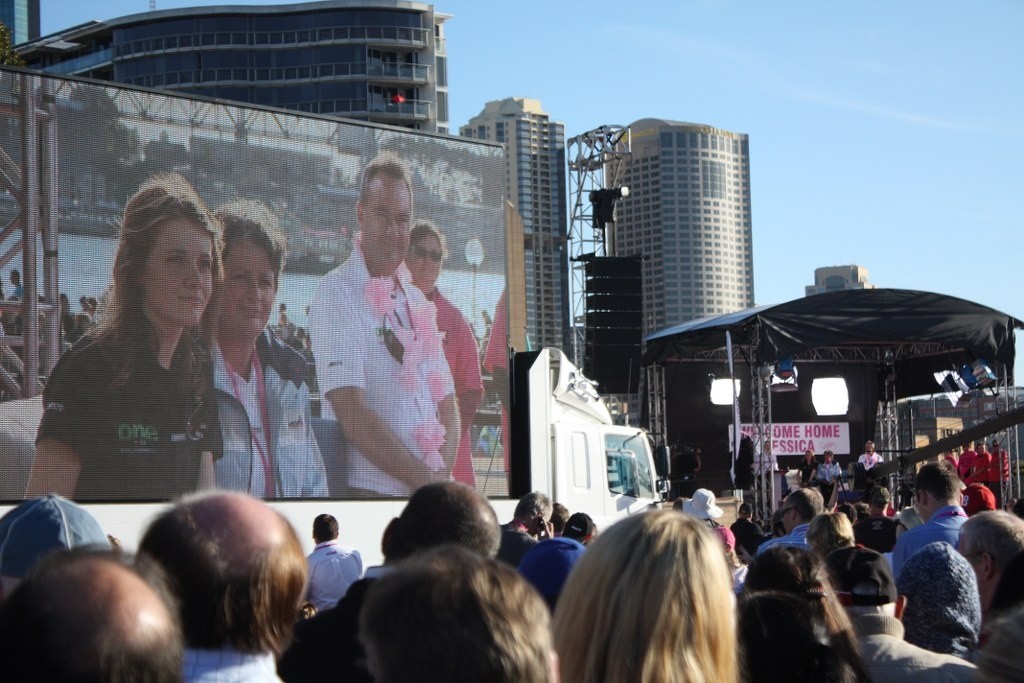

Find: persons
[0, 439, 1024, 682]
[310, 151, 463, 498]
[24, 172, 226, 502]
[480, 287, 509, 473]
[0, 271, 493, 392]
[208, 201, 330, 497]
[402, 220, 486, 488]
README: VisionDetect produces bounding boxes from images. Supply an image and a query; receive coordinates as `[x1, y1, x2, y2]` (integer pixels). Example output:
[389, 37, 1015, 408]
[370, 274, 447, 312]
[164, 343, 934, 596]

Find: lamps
[765, 352, 799, 391]
[933, 348, 998, 409]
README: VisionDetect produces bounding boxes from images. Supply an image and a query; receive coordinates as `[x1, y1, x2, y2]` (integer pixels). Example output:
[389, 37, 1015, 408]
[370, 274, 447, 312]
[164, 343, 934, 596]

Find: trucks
[0, 343, 673, 581]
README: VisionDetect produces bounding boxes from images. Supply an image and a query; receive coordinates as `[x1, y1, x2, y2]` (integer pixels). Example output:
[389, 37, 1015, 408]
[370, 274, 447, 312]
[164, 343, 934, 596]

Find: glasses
[411, 246, 441, 263]
[382, 313, 404, 365]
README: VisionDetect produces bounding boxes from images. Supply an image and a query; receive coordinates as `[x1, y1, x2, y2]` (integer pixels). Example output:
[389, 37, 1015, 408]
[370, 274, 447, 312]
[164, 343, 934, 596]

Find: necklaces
[220, 349, 276, 498]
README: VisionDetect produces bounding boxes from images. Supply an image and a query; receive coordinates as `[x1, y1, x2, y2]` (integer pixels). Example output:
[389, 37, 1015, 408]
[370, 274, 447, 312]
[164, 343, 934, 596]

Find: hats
[823, 544, 897, 607]
[0, 493, 112, 580]
[682, 488, 723, 520]
[518, 536, 591, 616]
[960, 483, 996, 514]
[738, 503, 754, 514]
